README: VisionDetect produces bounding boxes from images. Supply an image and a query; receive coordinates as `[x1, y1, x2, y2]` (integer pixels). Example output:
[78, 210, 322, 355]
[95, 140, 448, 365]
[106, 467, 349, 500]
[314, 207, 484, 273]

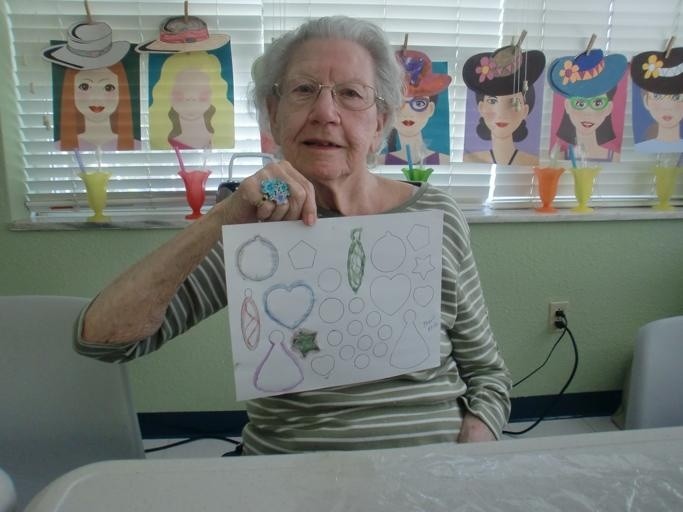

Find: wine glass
[567, 167, 602, 214]
[176, 168, 212, 220]
[76, 170, 113, 222]
[531, 166, 566, 214]
[649, 164, 683, 212]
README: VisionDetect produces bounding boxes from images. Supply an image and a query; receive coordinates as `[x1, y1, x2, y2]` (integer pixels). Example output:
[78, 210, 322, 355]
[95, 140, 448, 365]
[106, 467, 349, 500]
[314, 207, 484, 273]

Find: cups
[401, 166, 434, 181]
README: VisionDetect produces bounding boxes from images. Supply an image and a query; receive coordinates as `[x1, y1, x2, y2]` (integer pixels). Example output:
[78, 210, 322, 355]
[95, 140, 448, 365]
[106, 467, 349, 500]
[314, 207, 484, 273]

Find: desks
[25, 426, 682, 512]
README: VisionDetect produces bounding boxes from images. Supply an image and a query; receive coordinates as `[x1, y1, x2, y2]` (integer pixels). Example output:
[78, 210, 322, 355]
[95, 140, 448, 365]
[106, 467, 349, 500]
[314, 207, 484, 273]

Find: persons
[547, 47, 628, 163]
[68, 11, 513, 457]
[461, 44, 547, 165]
[54, 59, 142, 152]
[628, 47, 682, 156]
[374, 47, 449, 166]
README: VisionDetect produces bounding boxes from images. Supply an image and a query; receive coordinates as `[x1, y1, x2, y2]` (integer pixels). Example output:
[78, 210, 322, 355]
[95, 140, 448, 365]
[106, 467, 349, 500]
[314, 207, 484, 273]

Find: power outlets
[549, 301, 567, 333]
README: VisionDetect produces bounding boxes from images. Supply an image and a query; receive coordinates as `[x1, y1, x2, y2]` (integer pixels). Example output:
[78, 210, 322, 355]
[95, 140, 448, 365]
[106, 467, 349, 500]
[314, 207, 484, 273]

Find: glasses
[404, 97, 429, 111]
[570, 97, 608, 110]
[274, 76, 385, 111]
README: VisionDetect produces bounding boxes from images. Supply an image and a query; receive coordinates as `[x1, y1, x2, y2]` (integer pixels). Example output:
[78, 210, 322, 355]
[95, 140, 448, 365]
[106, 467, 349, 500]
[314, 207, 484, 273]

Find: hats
[40, 16, 232, 71]
[393, 46, 683, 99]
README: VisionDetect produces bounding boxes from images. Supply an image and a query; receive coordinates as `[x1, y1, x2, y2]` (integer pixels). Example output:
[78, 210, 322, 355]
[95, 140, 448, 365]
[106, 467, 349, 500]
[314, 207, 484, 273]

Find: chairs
[0, 295, 145, 512]
[625, 316, 683, 430]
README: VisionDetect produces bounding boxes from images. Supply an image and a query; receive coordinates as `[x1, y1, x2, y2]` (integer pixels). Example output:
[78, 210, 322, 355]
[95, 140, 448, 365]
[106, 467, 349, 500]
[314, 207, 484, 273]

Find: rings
[259, 177, 291, 206]
[255, 194, 267, 209]
